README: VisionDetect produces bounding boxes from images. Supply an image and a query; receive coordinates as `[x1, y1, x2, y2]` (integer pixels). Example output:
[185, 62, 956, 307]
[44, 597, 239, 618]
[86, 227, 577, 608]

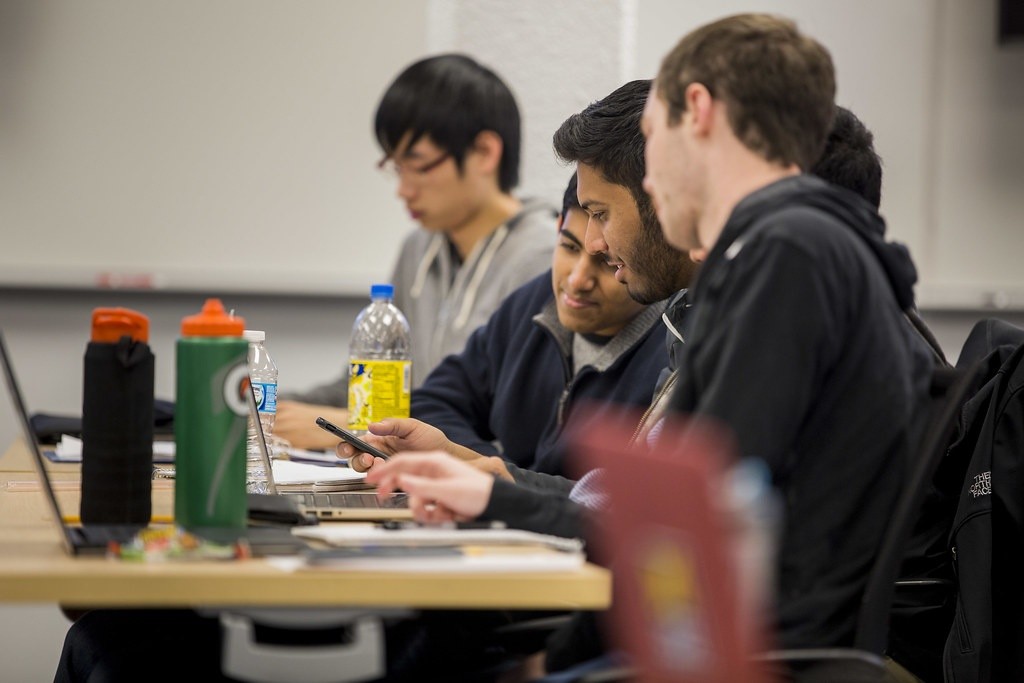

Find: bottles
[345, 284, 412, 436]
[242, 328, 276, 495]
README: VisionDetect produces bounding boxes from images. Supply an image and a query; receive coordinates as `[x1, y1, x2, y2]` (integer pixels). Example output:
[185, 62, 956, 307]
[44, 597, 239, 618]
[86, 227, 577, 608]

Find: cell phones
[315, 417, 389, 460]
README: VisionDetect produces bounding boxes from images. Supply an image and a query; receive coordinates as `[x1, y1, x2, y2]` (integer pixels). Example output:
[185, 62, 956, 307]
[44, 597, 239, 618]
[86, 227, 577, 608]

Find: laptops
[1, 332, 434, 558]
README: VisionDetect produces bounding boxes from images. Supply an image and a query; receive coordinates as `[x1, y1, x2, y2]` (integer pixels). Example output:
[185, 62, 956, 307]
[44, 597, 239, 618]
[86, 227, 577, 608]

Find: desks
[0, 441, 613, 611]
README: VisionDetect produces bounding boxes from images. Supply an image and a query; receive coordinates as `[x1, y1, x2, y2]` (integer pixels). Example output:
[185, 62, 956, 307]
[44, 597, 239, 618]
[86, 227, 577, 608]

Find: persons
[809, 103, 954, 372]
[337, 78, 704, 544]
[366, 14, 913, 683]
[272, 50, 563, 451]
[410, 165, 693, 481]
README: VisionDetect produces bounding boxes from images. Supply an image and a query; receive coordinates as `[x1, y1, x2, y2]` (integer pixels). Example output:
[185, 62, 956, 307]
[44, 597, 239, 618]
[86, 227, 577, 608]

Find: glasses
[377, 154, 452, 183]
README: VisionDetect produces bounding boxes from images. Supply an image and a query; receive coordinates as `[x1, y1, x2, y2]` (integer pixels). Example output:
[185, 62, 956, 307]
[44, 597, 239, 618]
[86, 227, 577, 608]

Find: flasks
[81, 307, 155, 530]
[170, 299, 251, 536]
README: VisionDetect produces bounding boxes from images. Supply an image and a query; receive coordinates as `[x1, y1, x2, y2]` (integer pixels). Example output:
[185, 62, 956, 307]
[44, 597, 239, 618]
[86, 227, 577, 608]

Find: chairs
[586, 316, 1024, 683]
